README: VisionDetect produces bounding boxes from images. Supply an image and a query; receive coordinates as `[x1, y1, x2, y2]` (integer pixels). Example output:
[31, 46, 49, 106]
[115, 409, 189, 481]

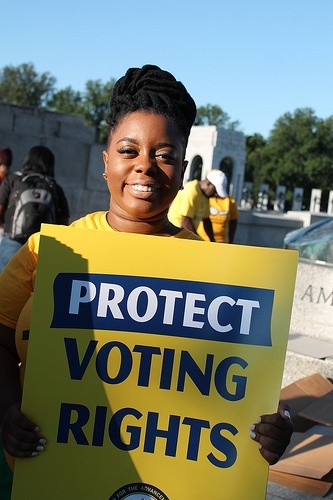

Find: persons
[0, 65, 296, 500]
[0, 145, 71, 243]
[168, 168, 227, 243]
[194, 197, 238, 244]
